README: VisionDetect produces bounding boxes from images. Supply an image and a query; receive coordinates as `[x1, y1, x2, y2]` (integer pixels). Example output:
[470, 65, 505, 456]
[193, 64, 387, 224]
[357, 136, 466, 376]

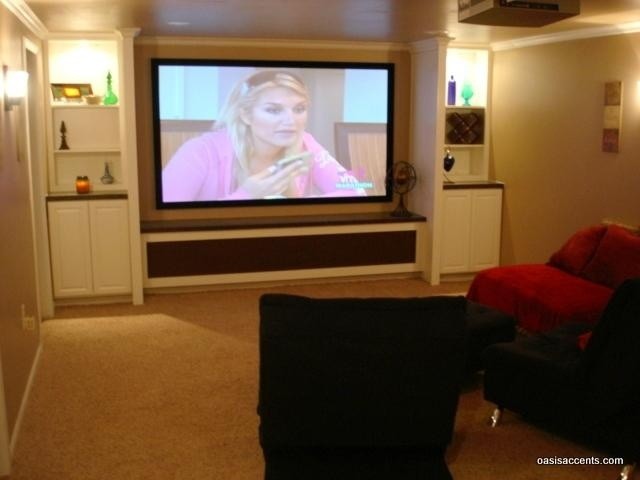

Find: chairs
[161, 119, 217, 170]
[257, 293, 469, 478]
[335, 123, 387, 196]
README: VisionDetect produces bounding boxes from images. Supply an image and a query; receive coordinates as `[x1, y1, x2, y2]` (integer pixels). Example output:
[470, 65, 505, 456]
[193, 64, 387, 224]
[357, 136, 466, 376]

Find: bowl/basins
[82, 94, 104, 105]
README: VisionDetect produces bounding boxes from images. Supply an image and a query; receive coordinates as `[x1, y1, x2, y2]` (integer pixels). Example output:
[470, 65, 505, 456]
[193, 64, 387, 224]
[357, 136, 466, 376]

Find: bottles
[447, 74, 457, 107]
[101, 162, 114, 184]
[103, 70, 118, 105]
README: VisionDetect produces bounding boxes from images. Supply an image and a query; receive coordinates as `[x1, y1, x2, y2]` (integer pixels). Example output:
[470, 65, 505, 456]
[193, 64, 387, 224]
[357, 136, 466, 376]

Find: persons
[161, 66, 370, 196]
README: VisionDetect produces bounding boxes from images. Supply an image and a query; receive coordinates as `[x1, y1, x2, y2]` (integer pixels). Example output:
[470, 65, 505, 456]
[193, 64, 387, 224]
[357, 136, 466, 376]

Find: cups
[75, 175, 91, 195]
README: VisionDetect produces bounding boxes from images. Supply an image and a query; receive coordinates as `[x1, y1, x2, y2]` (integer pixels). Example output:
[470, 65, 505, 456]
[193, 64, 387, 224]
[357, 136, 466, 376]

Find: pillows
[549, 226, 606, 277]
[585, 227, 640, 288]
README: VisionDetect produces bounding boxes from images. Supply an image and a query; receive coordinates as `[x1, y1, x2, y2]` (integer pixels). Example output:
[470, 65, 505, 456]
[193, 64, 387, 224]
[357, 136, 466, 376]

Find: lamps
[3, 65, 28, 112]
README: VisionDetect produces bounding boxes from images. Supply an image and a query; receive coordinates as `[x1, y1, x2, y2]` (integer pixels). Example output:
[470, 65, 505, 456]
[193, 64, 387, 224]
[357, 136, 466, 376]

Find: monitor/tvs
[151, 58, 395, 209]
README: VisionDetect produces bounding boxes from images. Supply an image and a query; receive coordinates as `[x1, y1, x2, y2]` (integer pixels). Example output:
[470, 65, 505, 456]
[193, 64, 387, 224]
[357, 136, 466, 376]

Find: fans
[391, 162, 417, 215]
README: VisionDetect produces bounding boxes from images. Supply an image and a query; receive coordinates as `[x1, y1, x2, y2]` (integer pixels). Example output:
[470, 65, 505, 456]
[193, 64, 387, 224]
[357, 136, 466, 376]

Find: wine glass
[461, 82, 475, 106]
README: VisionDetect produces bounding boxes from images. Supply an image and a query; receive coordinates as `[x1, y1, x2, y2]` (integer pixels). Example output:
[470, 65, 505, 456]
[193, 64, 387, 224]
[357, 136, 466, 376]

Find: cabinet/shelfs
[43, 39, 126, 192]
[48, 199, 131, 298]
[440, 104, 489, 182]
[443, 189, 503, 273]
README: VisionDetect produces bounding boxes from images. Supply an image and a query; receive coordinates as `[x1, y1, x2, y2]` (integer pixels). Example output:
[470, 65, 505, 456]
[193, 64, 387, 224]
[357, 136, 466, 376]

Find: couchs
[464, 222, 639, 347]
[484, 279, 640, 479]
